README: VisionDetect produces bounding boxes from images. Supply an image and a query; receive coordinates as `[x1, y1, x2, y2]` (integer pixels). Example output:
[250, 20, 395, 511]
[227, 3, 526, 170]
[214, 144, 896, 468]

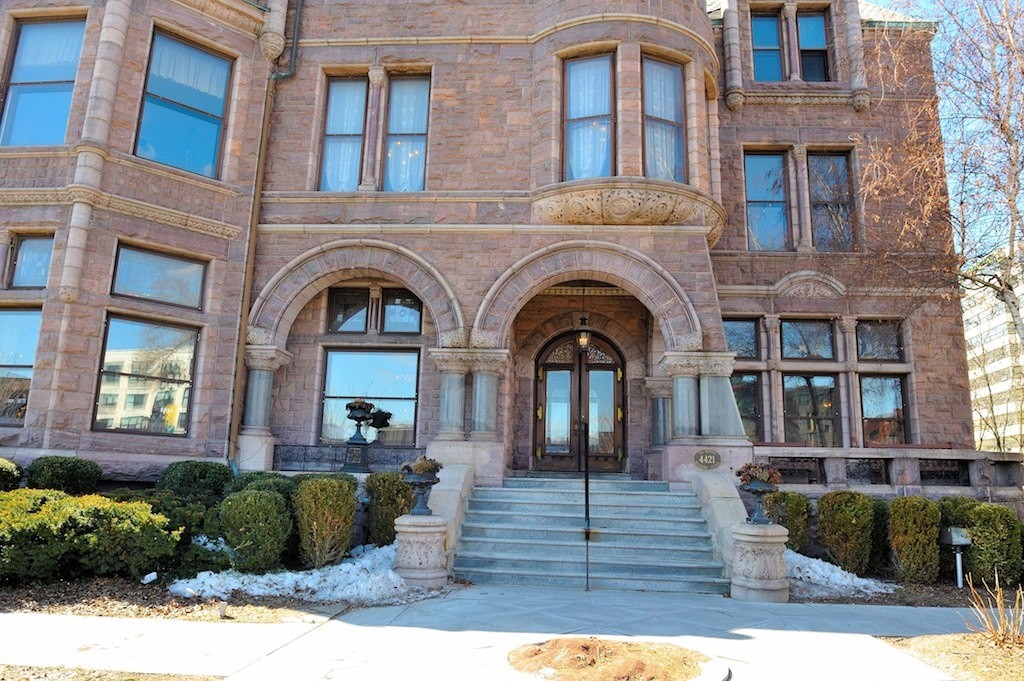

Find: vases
[402, 471, 440, 514]
[738, 478, 779, 524]
[347, 405, 373, 446]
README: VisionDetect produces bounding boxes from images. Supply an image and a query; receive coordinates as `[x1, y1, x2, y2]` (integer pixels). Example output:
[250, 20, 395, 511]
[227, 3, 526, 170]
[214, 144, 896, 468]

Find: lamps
[572, 288, 593, 352]
[944, 526, 973, 589]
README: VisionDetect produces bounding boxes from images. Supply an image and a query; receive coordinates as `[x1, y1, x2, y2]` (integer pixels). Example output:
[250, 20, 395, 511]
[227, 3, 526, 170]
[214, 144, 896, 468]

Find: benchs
[866, 440, 974, 485]
[752, 438, 819, 484]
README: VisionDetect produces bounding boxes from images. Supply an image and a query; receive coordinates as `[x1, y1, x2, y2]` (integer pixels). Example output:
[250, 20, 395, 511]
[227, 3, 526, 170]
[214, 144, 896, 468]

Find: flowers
[346, 397, 375, 411]
[400, 455, 443, 475]
[736, 457, 782, 486]
